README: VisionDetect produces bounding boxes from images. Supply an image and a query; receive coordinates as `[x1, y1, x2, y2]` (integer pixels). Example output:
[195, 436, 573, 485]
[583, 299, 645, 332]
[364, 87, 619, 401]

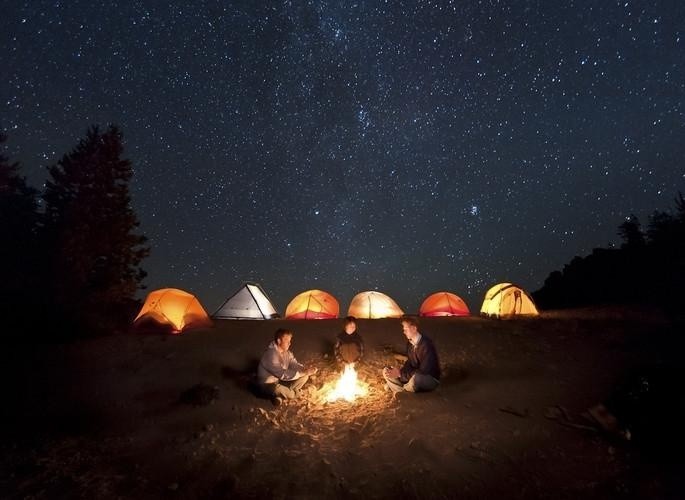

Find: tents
[480, 282, 540, 319]
[285, 289, 340, 320]
[212, 283, 281, 321]
[348, 291, 404, 320]
[132, 287, 212, 334]
[418, 291, 472, 317]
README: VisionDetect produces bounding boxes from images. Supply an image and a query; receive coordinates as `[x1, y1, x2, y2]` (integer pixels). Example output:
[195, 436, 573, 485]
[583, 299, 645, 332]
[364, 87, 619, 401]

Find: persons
[334, 316, 365, 371]
[380, 317, 441, 395]
[258, 328, 319, 405]
